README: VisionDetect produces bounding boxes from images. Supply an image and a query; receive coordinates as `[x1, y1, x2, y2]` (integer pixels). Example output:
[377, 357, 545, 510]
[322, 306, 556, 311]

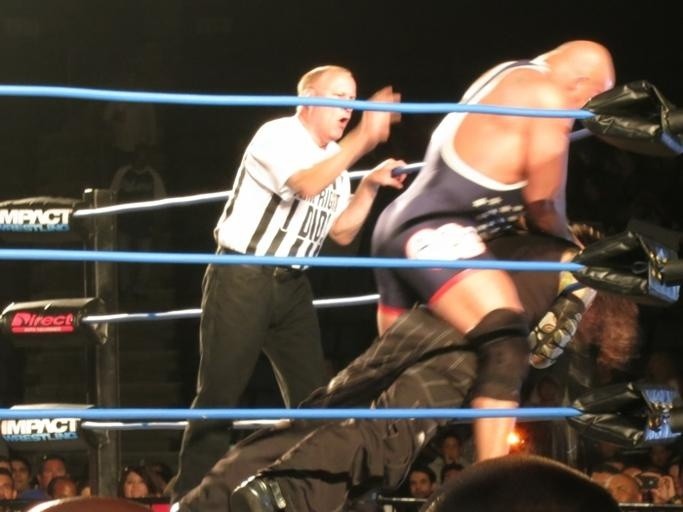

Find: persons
[2, 350, 682, 512]
[369, 35, 616, 464]
[168, 225, 643, 512]
[159, 64, 406, 503]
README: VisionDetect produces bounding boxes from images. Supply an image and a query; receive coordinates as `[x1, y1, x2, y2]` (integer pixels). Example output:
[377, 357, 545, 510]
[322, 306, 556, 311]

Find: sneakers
[229, 472, 287, 512]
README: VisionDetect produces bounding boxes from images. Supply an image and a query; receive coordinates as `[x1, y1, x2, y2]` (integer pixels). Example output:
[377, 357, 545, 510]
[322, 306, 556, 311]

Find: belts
[218, 246, 303, 282]
